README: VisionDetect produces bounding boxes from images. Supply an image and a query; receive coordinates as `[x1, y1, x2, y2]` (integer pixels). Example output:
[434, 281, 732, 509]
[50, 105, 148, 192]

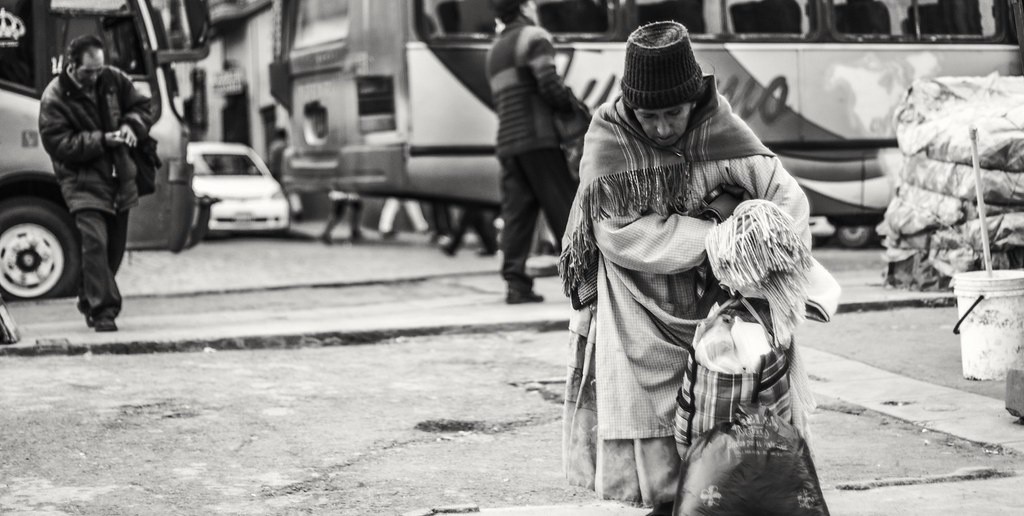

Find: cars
[183, 142, 292, 240]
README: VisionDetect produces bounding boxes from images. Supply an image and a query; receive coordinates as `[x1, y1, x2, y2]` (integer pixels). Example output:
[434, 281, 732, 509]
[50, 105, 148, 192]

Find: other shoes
[76, 301, 96, 328]
[92, 314, 118, 332]
[505, 286, 544, 306]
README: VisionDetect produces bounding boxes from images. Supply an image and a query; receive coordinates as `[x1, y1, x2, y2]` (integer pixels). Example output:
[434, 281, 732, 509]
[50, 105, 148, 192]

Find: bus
[1, 0, 214, 304]
[267, 0, 1024, 247]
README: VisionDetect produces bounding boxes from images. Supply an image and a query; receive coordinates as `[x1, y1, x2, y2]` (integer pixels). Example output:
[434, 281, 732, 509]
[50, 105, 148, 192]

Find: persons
[320, 190, 367, 246]
[268, 129, 289, 181]
[485, 0, 592, 304]
[437, 200, 495, 257]
[38, 36, 154, 332]
[378, 197, 431, 243]
[557, 20, 811, 516]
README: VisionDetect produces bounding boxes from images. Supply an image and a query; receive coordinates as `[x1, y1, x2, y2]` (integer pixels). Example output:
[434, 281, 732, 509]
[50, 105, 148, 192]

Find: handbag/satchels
[125, 120, 164, 198]
[555, 102, 593, 142]
[673, 298, 792, 464]
[675, 401, 831, 516]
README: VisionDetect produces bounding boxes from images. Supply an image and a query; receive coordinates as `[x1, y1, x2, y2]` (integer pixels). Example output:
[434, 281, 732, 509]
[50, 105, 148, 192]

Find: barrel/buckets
[953, 270, 1022, 380]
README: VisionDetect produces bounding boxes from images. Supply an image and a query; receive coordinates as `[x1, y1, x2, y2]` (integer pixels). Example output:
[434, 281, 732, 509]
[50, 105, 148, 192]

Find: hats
[621, 18, 703, 110]
